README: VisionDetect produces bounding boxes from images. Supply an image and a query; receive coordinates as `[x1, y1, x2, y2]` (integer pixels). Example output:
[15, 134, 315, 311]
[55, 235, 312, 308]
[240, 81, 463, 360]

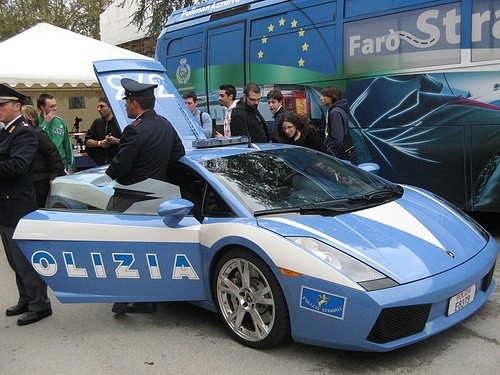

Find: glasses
[247, 96, 261, 101]
[97, 105, 105, 108]
[281, 124, 295, 131]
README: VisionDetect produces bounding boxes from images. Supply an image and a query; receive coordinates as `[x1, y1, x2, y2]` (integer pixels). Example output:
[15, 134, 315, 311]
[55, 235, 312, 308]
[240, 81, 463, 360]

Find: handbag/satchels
[337, 134, 358, 161]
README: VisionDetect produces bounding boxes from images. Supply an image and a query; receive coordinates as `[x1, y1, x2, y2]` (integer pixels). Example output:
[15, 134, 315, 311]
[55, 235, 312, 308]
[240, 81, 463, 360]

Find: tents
[0, 20, 156, 156]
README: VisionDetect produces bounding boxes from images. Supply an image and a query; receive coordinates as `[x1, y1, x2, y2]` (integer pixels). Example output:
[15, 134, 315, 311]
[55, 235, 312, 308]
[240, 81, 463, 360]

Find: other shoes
[112, 303, 159, 313]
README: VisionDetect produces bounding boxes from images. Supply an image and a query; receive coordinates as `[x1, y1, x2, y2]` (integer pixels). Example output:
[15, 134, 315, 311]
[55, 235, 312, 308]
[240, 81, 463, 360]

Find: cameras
[75, 117, 82, 122]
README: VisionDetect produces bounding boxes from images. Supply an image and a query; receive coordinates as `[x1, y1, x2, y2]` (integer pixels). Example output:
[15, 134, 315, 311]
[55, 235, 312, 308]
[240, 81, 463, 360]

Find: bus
[157, 0, 500, 211]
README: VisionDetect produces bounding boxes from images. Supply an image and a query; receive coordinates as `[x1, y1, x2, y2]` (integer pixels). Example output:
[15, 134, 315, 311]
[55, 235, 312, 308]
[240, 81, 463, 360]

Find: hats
[0, 83, 27, 104]
[120, 78, 158, 99]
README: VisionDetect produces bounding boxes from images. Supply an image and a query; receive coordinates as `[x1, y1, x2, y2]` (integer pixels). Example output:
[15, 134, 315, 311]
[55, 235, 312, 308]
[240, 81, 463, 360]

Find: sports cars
[11, 58, 499, 353]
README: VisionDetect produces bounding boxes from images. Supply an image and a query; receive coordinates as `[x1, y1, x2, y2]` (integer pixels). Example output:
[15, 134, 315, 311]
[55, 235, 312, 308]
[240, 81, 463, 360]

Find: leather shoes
[17, 310, 52, 326]
[6, 303, 29, 316]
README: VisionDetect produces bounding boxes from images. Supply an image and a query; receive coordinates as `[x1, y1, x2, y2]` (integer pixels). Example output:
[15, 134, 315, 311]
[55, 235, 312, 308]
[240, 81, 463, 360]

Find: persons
[266, 90, 291, 143]
[21, 104, 64, 208]
[85, 98, 122, 166]
[278, 113, 325, 153]
[103, 78, 186, 313]
[320, 86, 348, 158]
[35, 95, 76, 175]
[215, 85, 237, 137]
[182, 90, 212, 139]
[230, 83, 270, 143]
[0, 84, 53, 327]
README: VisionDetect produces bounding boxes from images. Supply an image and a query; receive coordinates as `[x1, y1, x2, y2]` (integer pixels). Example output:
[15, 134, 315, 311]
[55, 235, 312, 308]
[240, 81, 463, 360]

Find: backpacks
[200, 111, 216, 138]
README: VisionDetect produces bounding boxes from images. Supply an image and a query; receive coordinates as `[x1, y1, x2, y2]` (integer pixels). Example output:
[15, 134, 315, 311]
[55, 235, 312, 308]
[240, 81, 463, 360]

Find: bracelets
[96, 141, 100, 147]
